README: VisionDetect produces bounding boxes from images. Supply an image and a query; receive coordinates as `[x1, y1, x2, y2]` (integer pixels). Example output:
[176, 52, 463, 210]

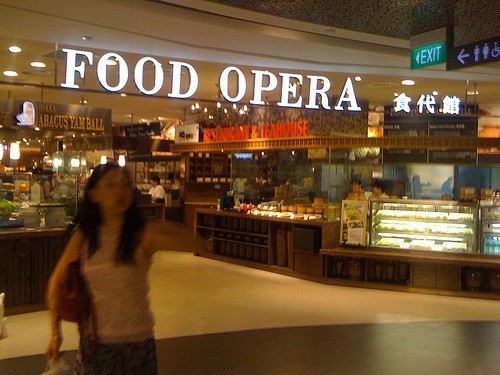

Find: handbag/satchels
[40, 352, 70, 375]
[61, 222, 90, 323]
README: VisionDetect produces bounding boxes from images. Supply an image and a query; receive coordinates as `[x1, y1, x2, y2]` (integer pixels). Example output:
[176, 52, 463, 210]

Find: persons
[256, 174, 275, 204]
[147, 176, 165, 203]
[34, 170, 57, 204]
[368, 182, 389, 218]
[244, 176, 259, 204]
[345, 180, 372, 200]
[166, 173, 181, 189]
[46, 161, 208, 375]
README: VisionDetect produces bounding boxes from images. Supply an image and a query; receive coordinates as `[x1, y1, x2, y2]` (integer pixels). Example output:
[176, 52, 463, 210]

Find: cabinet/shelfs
[184, 152, 231, 184]
[193, 208, 340, 278]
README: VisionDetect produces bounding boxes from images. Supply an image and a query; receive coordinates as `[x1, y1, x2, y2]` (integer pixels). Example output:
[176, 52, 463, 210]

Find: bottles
[483, 236, 500, 254]
